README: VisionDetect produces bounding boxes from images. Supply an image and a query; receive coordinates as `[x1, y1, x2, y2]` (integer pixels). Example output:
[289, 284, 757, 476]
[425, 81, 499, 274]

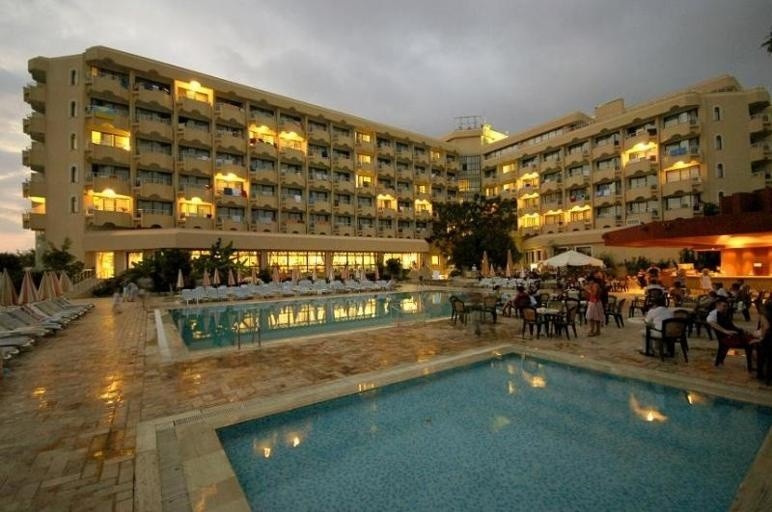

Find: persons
[492, 264, 771, 374]
[112, 280, 138, 313]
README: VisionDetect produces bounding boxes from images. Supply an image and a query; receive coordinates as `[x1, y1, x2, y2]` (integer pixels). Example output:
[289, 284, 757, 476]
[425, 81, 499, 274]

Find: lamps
[0, 296, 95, 361]
[181, 278, 396, 305]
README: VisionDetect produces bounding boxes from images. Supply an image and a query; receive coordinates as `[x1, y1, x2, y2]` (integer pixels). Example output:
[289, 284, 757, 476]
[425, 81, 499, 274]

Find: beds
[542, 248, 605, 268]
[176, 269, 185, 299]
[202, 264, 380, 289]
[0, 268, 75, 307]
